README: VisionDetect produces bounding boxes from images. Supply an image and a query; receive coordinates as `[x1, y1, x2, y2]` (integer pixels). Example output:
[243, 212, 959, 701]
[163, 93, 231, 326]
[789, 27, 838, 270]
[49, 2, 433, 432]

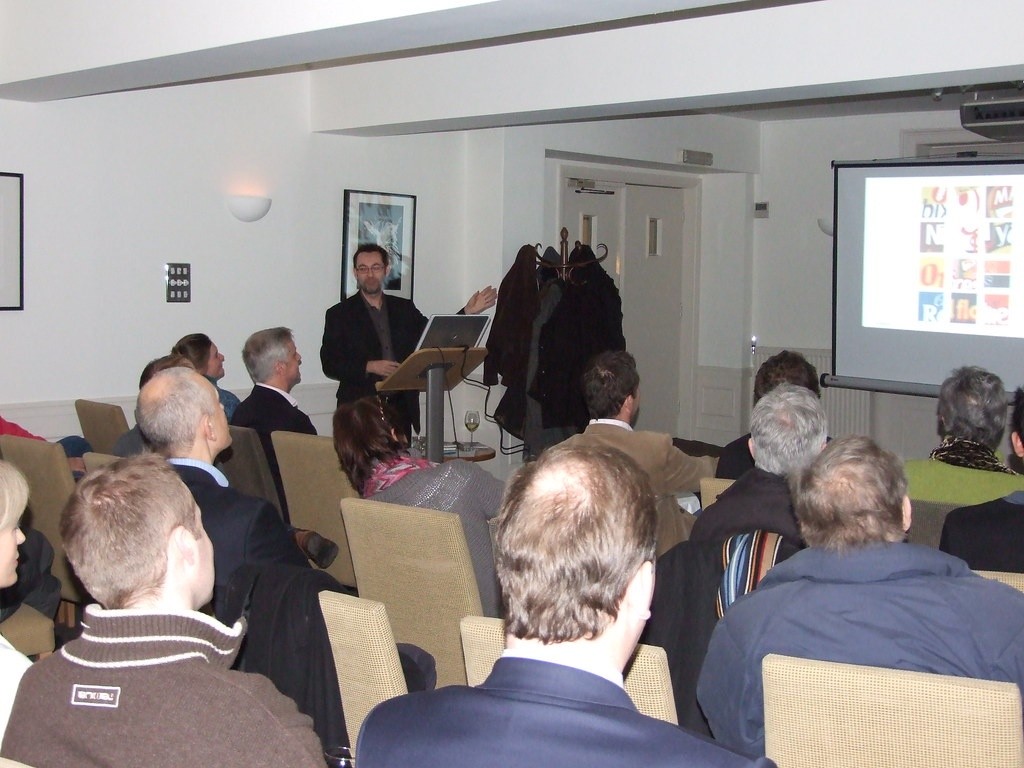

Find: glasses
[355, 264, 385, 274]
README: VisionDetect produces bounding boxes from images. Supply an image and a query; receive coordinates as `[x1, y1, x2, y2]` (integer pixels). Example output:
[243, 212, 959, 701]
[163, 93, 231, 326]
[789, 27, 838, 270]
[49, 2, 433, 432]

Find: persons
[320, 243, 497, 448]
[0, 326, 351, 768]
[903, 365, 1024, 504]
[691, 382, 826, 550]
[0, 449, 332, 768]
[939, 386, 1024, 574]
[538, 350, 721, 559]
[356, 433, 778, 768]
[716, 350, 833, 480]
[696, 436, 1024, 763]
[332, 392, 507, 620]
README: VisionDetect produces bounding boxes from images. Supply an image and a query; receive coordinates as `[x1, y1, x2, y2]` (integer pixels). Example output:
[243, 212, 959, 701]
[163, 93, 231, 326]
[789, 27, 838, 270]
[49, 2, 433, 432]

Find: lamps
[817, 218, 834, 236]
[226, 193, 272, 222]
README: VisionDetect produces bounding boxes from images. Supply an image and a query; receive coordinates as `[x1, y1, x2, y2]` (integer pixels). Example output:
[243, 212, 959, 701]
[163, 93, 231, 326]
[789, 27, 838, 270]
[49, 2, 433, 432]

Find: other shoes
[301, 532, 338, 570]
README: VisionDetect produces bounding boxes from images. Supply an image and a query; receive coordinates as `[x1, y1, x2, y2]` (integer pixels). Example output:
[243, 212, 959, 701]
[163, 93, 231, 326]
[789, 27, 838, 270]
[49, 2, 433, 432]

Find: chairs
[0, 399, 1024, 768]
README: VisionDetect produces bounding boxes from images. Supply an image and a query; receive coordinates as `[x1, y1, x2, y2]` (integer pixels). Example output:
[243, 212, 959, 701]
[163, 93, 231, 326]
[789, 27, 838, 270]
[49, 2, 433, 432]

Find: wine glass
[465, 412, 480, 448]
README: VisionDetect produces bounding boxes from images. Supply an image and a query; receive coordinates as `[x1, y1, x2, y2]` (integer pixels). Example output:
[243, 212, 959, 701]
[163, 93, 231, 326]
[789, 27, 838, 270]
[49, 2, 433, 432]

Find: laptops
[416, 314, 491, 351]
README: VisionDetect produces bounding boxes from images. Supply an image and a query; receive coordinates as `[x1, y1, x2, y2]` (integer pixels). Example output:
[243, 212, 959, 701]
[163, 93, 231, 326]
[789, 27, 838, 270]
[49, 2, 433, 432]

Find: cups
[412, 436, 425, 454]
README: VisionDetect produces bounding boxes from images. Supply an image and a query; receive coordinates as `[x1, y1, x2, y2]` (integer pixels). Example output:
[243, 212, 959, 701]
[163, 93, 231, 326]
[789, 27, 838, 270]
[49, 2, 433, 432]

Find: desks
[441, 441, 496, 464]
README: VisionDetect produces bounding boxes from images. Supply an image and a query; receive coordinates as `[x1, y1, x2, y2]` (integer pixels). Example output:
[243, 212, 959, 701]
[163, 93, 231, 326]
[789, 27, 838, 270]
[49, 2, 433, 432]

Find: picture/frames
[0, 172, 24, 311]
[341, 189, 417, 301]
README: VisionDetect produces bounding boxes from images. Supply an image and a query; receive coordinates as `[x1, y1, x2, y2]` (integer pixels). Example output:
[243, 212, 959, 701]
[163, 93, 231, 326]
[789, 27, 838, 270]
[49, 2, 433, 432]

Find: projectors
[959, 96, 1024, 142]
